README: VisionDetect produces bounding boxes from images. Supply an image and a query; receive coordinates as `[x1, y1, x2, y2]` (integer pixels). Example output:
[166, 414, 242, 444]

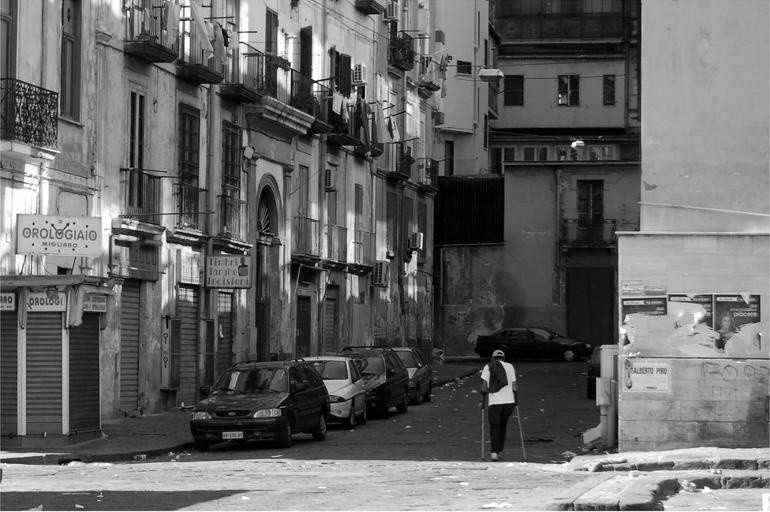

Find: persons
[478, 349, 519, 461]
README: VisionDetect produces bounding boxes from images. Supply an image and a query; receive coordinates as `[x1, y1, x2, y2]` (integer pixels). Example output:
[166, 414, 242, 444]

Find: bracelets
[480, 400, 485, 403]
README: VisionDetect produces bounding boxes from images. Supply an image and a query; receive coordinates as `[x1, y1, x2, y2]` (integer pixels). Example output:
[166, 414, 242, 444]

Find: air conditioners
[370, 260, 391, 287]
[324, 169, 338, 192]
[383, 2, 400, 23]
[352, 63, 369, 86]
[408, 232, 425, 252]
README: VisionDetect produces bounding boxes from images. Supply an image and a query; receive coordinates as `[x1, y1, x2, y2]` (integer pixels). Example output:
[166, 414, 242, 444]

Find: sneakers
[491, 452, 508, 461]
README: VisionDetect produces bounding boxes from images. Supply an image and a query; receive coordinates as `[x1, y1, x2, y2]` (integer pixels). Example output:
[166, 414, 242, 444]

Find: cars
[292, 355, 368, 430]
[384, 346, 432, 406]
[471, 327, 593, 362]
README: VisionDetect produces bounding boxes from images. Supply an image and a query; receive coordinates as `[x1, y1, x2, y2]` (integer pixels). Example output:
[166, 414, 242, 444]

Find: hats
[492, 350, 505, 357]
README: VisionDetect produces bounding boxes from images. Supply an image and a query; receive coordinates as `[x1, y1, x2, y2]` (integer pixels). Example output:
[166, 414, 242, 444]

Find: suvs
[190, 356, 331, 447]
[340, 343, 409, 421]
